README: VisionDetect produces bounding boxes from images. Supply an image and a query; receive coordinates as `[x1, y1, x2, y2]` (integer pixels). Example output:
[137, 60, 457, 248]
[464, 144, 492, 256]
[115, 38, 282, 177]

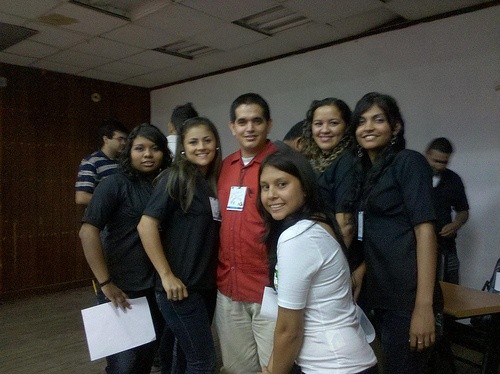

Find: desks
[439, 280, 500, 374]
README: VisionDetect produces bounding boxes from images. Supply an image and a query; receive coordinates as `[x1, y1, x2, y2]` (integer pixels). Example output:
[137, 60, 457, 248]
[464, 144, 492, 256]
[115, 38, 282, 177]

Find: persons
[165, 103, 200, 162]
[298, 96, 373, 252]
[350, 92, 443, 374]
[281, 120, 309, 155]
[423, 137, 469, 287]
[211, 94, 280, 374]
[256, 147, 380, 374]
[74, 119, 129, 307]
[137, 116, 223, 374]
[78, 121, 175, 374]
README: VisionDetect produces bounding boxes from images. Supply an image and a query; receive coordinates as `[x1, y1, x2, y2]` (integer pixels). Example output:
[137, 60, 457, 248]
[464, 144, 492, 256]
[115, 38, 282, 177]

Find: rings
[173, 296, 178, 298]
[417, 342, 423, 345]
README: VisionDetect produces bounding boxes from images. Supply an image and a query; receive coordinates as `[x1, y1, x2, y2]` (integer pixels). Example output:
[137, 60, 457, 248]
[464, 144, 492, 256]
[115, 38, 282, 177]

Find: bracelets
[96, 280, 112, 288]
